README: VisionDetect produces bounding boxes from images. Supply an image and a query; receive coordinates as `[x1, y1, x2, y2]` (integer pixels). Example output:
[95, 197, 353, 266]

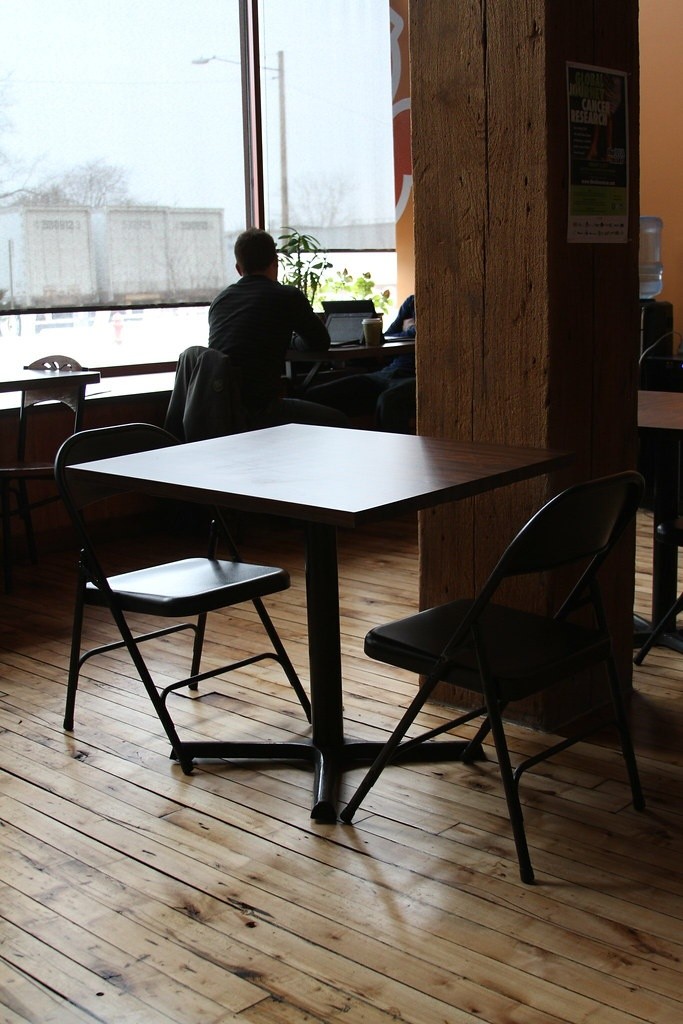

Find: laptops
[324, 313, 374, 347]
[320, 299, 404, 342]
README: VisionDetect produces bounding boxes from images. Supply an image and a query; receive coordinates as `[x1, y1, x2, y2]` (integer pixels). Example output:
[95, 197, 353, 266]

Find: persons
[208, 227, 348, 428]
[305, 294, 416, 433]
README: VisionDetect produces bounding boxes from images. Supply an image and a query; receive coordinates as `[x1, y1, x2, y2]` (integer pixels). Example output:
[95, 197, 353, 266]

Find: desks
[66, 422, 580, 824]
[633, 391, 683, 652]
[0, 369, 101, 393]
[283, 338, 415, 398]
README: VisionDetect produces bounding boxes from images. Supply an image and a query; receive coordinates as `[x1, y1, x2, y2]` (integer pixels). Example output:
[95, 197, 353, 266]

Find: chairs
[52, 421, 314, 775]
[337, 471, 647, 886]
[0, 356, 90, 596]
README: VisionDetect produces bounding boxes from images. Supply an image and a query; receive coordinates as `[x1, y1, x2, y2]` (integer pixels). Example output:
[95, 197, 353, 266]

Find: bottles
[639, 217, 663, 299]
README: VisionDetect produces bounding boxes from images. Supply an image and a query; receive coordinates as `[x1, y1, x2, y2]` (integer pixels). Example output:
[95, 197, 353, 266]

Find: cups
[362, 318, 383, 346]
